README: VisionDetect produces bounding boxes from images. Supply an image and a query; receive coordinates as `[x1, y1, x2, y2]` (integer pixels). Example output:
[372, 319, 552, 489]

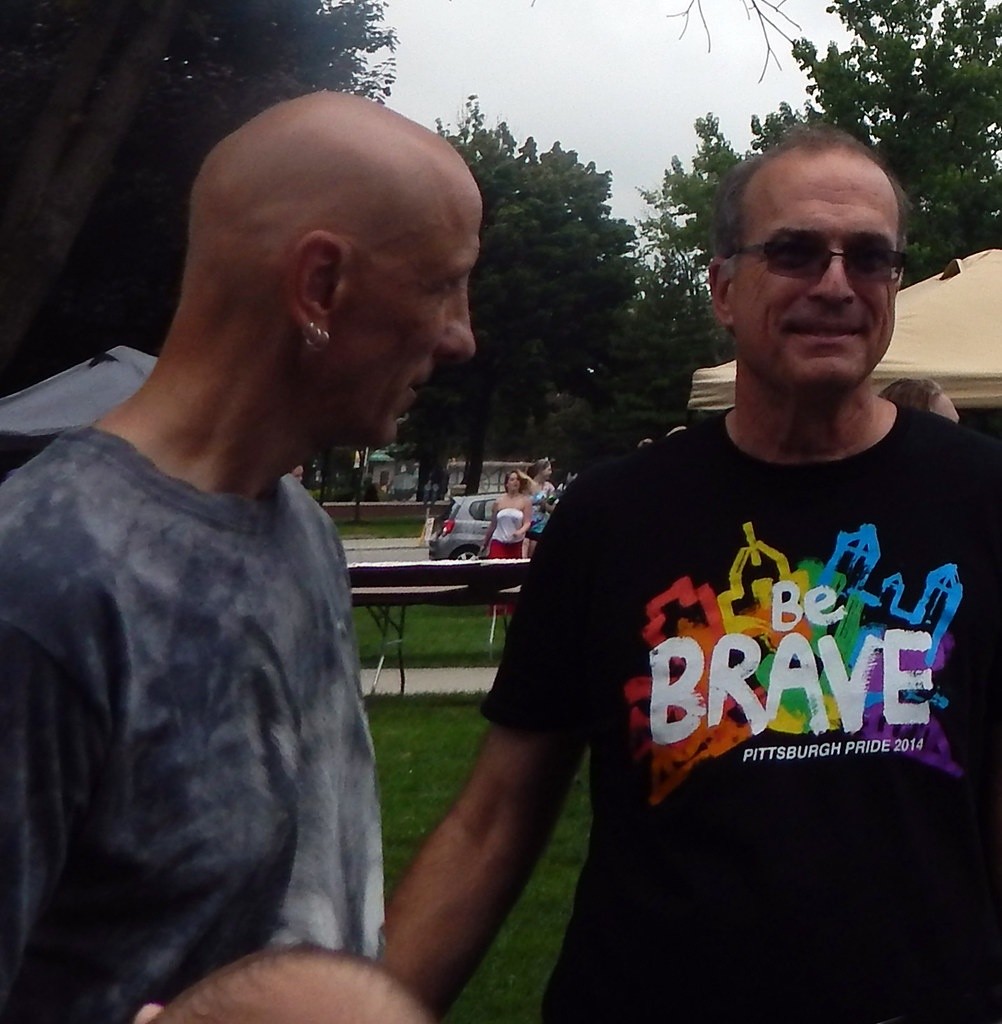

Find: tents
[685, 246, 1002, 411]
[0, 344, 160, 459]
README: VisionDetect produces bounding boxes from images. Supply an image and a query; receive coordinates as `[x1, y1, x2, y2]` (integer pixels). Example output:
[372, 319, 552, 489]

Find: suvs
[429, 491, 507, 561]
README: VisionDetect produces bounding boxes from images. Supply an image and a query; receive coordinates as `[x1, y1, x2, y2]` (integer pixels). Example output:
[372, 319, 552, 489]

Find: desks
[346, 558, 530, 587]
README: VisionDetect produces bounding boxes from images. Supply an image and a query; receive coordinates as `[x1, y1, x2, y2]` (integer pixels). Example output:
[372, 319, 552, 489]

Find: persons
[481, 470, 532, 615]
[1, 88, 485, 1024]
[291, 465, 303, 483]
[133, 939, 438, 1023]
[519, 457, 555, 558]
[376, 126, 1002, 1023]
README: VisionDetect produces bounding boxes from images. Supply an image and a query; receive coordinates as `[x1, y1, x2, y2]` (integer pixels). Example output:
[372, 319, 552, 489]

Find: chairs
[339, 585, 531, 695]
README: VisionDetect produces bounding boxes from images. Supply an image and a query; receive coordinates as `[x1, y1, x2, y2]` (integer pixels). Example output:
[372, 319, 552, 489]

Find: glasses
[735, 235, 912, 286]
